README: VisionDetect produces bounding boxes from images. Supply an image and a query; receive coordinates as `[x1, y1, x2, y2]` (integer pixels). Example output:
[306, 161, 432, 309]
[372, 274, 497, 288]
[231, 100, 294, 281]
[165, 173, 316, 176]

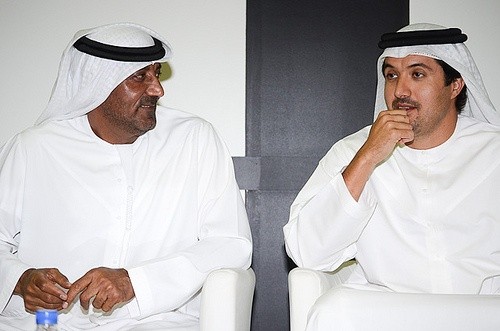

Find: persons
[283, 19, 500, 331]
[1, 22, 252, 331]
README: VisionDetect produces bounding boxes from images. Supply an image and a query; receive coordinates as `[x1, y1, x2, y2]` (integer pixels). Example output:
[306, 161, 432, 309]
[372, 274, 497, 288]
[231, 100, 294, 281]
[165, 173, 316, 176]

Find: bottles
[34, 309, 60, 331]
